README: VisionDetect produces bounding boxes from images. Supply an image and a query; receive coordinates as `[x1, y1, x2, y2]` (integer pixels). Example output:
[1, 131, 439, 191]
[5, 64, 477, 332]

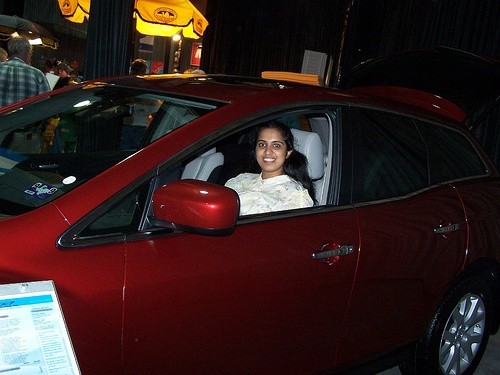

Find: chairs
[180, 147, 223, 181]
[291, 129, 328, 203]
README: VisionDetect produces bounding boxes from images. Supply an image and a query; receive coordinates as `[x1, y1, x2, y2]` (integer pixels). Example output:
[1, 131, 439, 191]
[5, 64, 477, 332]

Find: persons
[225, 122, 319, 218]
[0, 37, 301, 154]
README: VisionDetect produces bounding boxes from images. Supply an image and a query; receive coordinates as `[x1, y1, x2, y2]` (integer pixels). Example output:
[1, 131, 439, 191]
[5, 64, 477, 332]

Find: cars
[0, 46, 500, 375]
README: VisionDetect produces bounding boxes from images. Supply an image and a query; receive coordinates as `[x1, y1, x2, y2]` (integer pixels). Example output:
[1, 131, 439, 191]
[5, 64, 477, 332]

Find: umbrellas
[58, 0, 208, 39]
[0, 15, 60, 50]
